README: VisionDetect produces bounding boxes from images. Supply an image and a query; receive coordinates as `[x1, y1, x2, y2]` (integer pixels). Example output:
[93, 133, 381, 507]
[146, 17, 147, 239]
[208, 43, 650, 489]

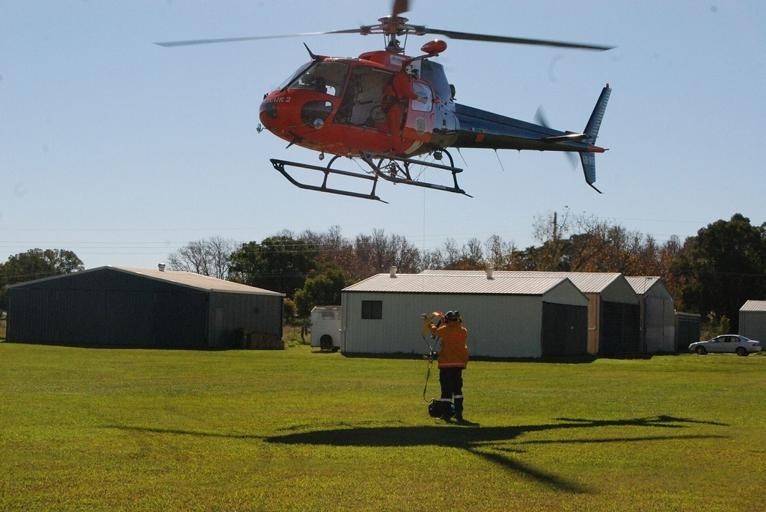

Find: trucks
[311, 304, 345, 353]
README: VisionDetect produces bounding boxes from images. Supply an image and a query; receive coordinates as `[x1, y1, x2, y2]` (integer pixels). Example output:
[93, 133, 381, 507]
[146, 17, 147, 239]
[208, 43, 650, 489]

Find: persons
[428, 309, 469, 420]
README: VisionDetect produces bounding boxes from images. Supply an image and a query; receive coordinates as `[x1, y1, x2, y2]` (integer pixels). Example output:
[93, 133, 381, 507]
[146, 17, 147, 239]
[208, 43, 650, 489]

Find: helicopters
[153, 0, 618, 204]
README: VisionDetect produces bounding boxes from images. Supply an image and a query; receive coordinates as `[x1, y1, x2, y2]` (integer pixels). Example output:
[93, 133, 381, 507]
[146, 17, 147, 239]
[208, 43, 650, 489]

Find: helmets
[444, 311, 462, 324]
[405, 64, 419, 79]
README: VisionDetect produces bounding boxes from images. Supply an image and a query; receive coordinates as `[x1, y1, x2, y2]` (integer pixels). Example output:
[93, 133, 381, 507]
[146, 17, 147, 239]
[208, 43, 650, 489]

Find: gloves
[415, 96, 428, 103]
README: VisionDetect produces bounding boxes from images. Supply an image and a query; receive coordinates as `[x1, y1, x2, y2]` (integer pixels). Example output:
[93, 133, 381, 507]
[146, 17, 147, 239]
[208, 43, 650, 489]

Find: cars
[689, 333, 761, 357]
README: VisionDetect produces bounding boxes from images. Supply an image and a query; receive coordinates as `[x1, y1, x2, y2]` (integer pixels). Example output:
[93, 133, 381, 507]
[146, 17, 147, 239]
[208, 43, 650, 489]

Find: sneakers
[396, 153, 409, 158]
